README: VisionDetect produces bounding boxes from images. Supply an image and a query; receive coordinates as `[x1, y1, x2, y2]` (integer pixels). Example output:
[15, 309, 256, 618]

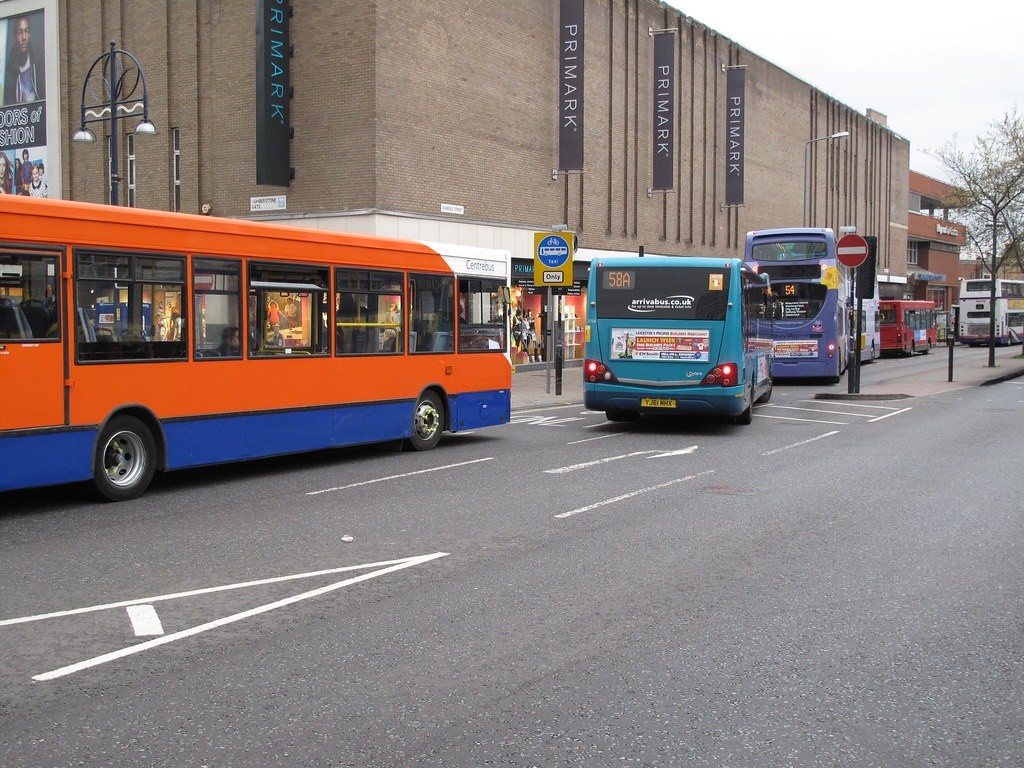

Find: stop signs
[835, 233, 867, 269]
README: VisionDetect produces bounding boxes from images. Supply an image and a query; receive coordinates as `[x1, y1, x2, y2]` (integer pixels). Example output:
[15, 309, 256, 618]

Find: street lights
[801, 131, 849, 225]
[68, 41, 157, 206]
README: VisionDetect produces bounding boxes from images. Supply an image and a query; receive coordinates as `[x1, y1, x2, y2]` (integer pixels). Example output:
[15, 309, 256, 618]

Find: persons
[0, 149, 47, 198]
[458, 304, 468, 325]
[512, 308, 540, 362]
[6, 15, 42, 103]
[215, 326, 240, 356]
[389, 303, 401, 324]
[285, 298, 298, 329]
[269, 301, 285, 345]
[155, 305, 182, 340]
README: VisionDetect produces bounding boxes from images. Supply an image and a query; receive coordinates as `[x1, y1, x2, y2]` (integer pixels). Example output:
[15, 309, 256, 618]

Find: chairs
[394, 329, 449, 353]
[0, 296, 150, 342]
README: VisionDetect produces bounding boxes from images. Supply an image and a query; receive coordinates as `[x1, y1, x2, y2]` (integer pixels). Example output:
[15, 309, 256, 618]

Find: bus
[0, 191, 514, 502]
[880, 300, 939, 357]
[584, 257, 775, 426]
[958, 278, 1024, 346]
[742, 228, 881, 382]
